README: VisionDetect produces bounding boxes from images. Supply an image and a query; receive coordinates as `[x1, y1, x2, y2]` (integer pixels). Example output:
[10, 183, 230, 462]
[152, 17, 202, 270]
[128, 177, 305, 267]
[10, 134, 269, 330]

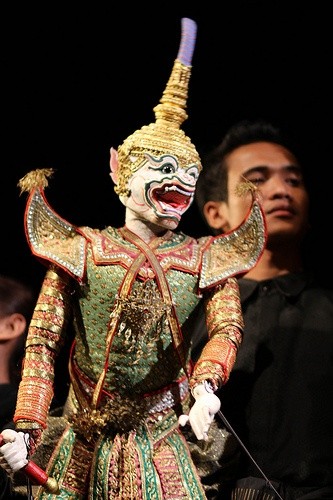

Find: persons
[0, 18, 266, 500]
[182, 122, 333, 500]
[0, 275, 35, 500]
